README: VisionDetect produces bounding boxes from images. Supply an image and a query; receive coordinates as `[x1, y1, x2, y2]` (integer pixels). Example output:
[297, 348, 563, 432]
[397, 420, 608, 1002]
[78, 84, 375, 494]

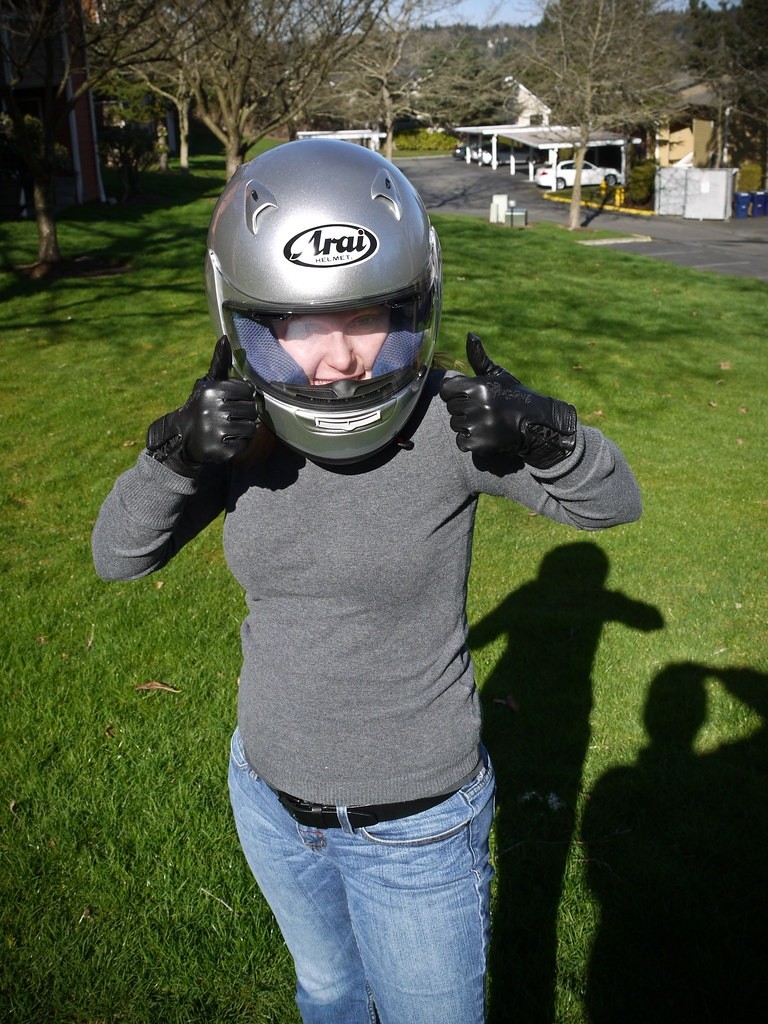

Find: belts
[268, 787, 458, 830]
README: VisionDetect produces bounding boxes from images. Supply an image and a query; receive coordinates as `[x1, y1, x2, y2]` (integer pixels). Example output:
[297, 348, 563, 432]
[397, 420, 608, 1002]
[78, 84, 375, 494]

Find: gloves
[441, 332, 577, 470]
[146, 335, 259, 478]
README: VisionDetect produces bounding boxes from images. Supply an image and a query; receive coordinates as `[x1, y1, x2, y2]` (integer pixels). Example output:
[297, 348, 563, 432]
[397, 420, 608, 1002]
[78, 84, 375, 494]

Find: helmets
[206, 138, 442, 466]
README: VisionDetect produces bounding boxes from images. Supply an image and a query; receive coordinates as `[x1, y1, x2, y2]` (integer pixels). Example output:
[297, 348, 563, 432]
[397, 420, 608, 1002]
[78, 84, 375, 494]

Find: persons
[89, 137, 642, 1024]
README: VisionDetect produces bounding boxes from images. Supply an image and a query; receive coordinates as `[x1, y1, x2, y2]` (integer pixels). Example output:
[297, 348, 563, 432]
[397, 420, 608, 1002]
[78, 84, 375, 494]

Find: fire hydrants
[599, 182, 607, 196]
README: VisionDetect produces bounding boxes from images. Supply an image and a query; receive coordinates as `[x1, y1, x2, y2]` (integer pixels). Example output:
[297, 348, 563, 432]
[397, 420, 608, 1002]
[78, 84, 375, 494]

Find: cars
[453, 141, 485, 157]
[472, 143, 541, 165]
[535, 159, 622, 190]
